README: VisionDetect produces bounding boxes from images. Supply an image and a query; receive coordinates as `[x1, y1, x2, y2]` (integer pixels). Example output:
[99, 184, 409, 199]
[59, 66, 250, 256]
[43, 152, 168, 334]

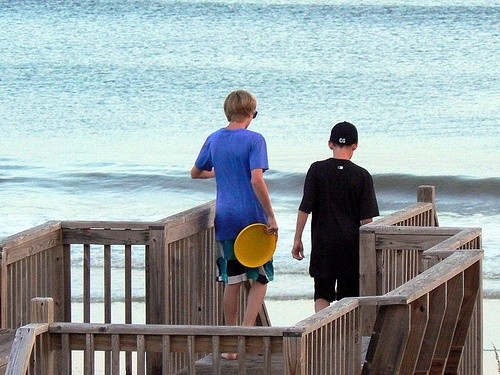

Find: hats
[331, 121, 358, 144]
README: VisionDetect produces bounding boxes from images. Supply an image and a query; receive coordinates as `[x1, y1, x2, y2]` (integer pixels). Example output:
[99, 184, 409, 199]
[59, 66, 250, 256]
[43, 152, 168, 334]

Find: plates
[234, 224, 277, 269]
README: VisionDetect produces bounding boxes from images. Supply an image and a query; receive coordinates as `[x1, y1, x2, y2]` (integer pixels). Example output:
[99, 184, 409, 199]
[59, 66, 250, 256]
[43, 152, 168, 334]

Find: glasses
[251, 111, 258, 118]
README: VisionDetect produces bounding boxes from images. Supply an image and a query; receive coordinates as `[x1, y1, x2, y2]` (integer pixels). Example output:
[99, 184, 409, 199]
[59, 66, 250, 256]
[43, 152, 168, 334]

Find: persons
[190, 89, 280, 361]
[291, 120, 381, 314]
